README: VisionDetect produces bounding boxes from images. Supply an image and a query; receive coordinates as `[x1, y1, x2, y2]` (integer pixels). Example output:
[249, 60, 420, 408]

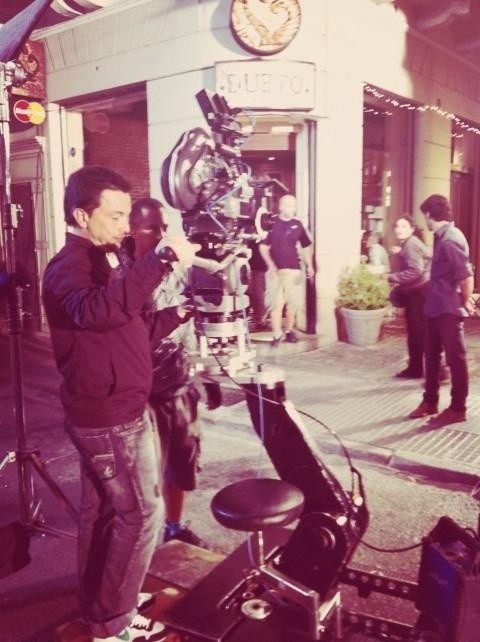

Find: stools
[214, 479, 305, 611]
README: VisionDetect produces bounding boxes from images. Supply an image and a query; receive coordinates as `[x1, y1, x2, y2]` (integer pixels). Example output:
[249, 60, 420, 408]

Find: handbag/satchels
[389, 286, 403, 309]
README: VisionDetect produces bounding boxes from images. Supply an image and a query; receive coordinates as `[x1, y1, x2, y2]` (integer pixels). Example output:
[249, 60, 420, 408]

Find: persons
[380, 214, 450, 378]
[258, 193, 315, 345]
[119, 197, 209, 551]
[38, 165, 203, 642]
[362, 230, 391, 273]
[409, 193, 474, 427]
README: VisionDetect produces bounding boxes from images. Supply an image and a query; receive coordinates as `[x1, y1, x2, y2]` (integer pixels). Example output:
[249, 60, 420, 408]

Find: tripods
[0, 137, 81, 539]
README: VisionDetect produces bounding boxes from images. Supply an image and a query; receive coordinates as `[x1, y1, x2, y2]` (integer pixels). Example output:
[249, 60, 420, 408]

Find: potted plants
[334, 254, 392, 346]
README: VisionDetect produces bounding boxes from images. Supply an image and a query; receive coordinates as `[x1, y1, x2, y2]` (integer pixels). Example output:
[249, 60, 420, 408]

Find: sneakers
[164, 521, 208, 549]
[78, 587, 171, 642]
[408, 398, 438, 420]
[394, 367, 451, 387]
[271, 331, 297, 345]
[429, 407, 467, 426]
